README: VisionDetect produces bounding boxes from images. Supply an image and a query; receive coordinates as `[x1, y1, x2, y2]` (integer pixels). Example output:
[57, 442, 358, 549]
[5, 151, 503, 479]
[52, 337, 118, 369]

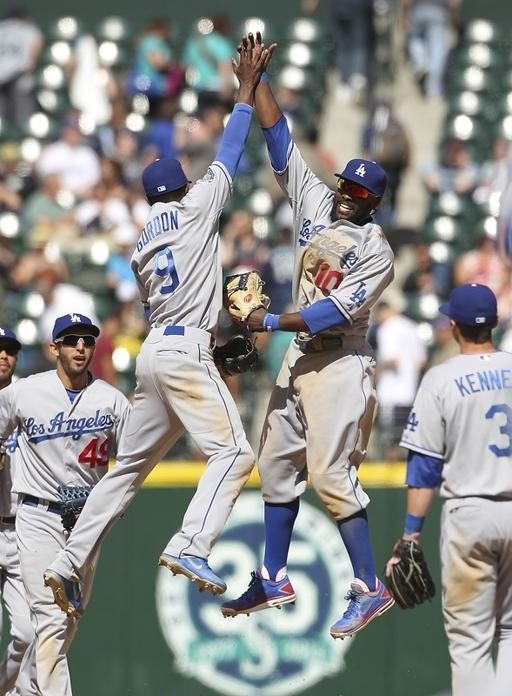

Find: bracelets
[402, 512, 429, 539]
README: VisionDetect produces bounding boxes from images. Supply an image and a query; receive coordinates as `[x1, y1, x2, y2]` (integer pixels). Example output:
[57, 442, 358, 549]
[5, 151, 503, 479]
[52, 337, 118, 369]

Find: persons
[0, 327, 38, 693]
[0, 0, 512, 466]
[221, 29, 397, 641]
[0, 307, 137, 694]
[378, 279, 512, 695]
[42, 30, 269, 623]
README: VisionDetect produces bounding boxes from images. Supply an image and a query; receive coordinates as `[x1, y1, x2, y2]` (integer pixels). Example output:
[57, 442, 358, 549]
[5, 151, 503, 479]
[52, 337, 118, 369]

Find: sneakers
[221, 563, 297, 619]
[328, 577, 395, 641]
[43, 566, 86, 620]
[156, 548, 227, 596]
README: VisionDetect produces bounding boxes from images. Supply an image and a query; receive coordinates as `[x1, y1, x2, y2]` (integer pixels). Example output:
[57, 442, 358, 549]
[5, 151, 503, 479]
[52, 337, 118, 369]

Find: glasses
[0, 342, 20, 356]
[53, 332, 97, 347]
[335, 176, 376, 199]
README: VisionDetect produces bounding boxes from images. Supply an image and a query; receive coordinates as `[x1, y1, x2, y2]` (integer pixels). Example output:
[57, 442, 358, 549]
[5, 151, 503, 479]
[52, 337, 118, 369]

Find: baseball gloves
[213, 334, 257, 377]
[223, 272, 273, 333]
[384, 540, 436, 612]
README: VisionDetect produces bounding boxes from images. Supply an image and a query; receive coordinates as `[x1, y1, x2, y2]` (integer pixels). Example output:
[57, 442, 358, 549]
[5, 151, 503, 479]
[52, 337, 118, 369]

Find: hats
[334, 159, 389, 200]
[51, 313, 100, 342]
[0, 326, 22, 351]
[140, 158, 192, 197]
[439, 283, 497, 327]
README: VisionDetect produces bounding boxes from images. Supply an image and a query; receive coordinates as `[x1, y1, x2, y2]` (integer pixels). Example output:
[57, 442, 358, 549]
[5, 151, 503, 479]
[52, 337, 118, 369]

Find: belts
[163, 325, 185, 336]
[22, 494, 66, 518]
[299, 337, 343, 354]
[0, 516, 16, 525]
